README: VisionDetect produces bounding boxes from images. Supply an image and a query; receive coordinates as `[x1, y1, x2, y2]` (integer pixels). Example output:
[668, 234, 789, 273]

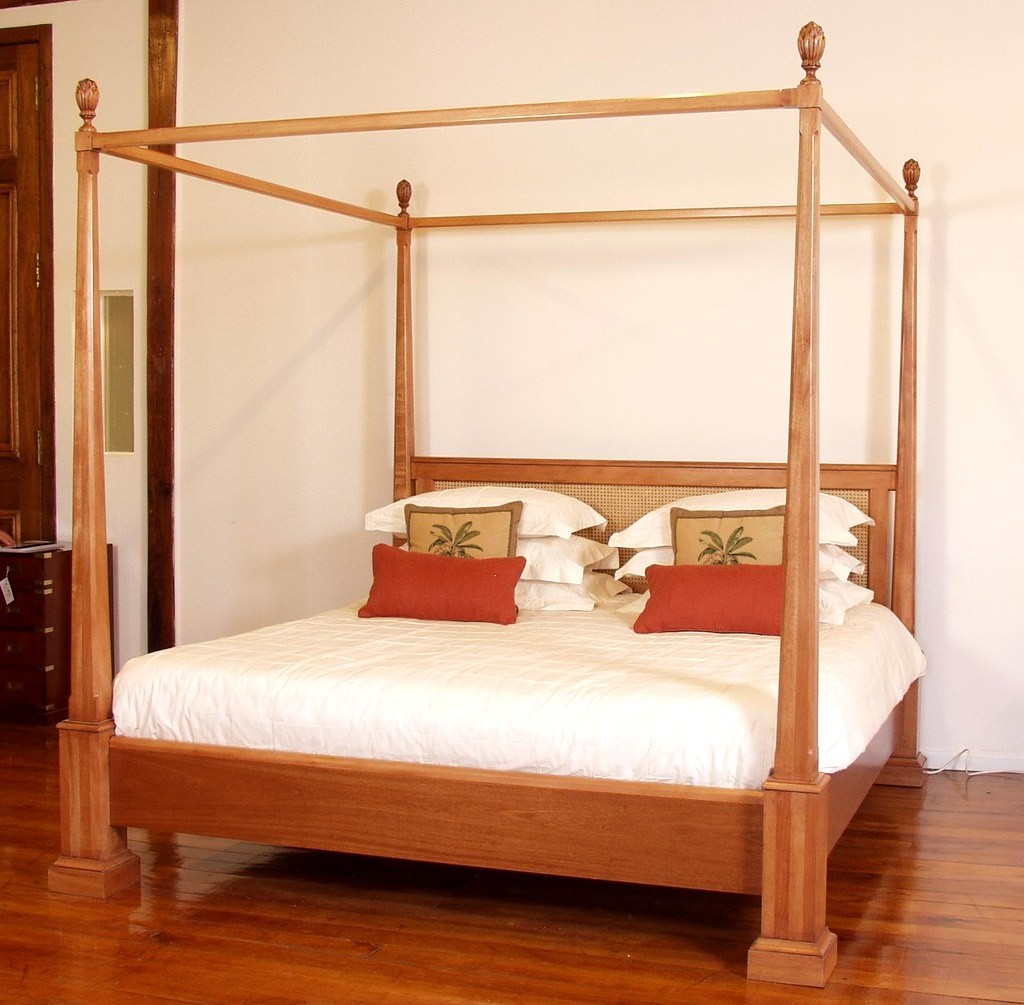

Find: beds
[47, 19, 926, 988]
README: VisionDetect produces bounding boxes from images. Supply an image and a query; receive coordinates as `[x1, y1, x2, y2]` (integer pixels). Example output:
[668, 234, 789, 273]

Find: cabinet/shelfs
[0, 542, 114, 726]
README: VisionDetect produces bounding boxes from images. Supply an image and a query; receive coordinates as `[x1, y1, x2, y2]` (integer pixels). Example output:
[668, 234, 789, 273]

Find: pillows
[669, 505, 786, 564]
[514, 572, 634, 613]
[363, 486, 607, 540]
[615, 580, 874, 625]
[404, 500, 523, 559]
[608, 488, 876, 553]
[358, 543, 526, 626]
[615, 544, 864, 582]
[399, 535, 619, 584]
[634, 564, 781, 637]
